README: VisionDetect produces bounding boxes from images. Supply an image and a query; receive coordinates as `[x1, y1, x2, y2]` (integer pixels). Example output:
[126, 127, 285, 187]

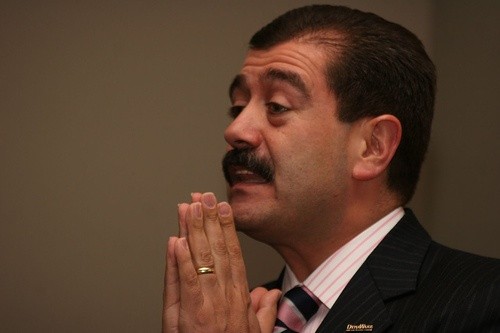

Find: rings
[196, 267, 214, 274]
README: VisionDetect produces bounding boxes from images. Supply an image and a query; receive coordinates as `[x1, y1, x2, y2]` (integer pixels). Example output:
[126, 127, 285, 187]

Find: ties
[272, 285, 323, 333]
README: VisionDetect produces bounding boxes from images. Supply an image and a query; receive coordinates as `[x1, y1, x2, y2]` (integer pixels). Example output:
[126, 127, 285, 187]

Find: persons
[163, 5, 500, 333]
